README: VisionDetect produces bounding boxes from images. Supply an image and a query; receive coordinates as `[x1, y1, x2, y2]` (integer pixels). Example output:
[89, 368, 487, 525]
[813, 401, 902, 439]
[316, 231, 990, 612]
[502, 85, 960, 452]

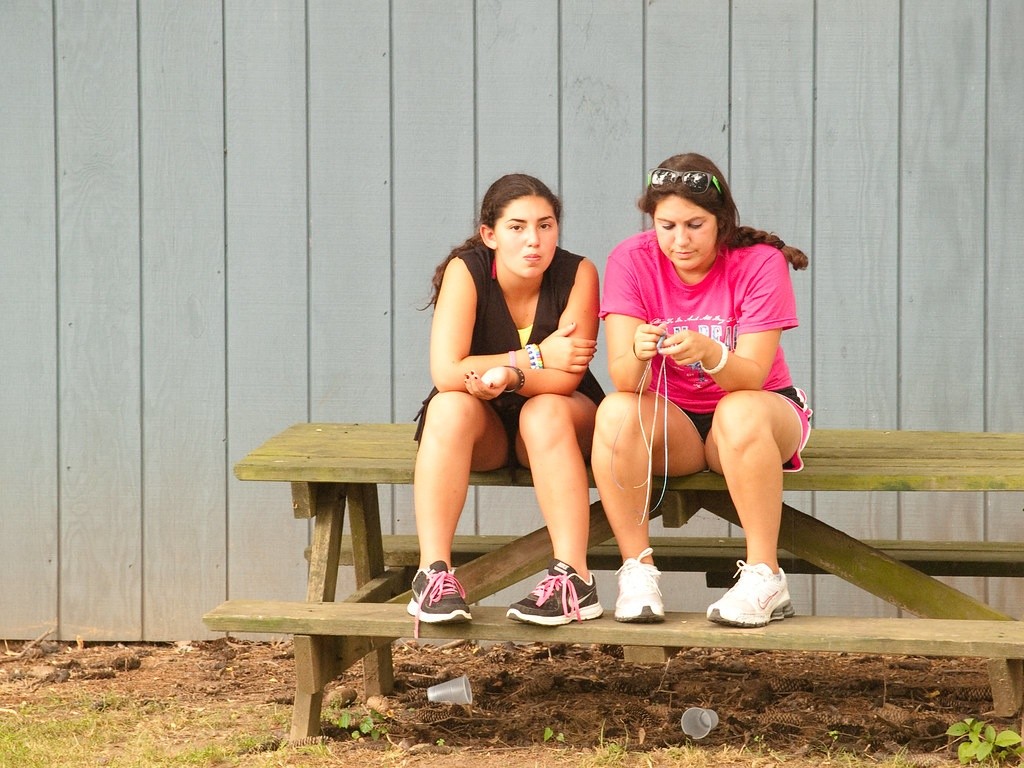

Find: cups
[682, 708, 719, 738]
[426, 675, 474, 705]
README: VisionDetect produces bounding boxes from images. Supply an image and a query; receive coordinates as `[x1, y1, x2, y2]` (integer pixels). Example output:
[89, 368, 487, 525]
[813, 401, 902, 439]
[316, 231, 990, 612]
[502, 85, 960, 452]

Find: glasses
[648, 168, 725, 194]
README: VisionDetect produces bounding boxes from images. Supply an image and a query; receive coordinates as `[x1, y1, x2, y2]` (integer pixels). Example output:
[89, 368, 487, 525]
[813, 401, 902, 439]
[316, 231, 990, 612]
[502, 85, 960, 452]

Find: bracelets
[524, 342, 544, 370]
[700, 336, 729, 375]
[508, 350, 516, 367]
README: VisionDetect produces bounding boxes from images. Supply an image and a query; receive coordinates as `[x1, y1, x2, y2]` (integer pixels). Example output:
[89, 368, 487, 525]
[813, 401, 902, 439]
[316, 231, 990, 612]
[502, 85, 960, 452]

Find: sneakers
[506, 560, 604, 628]
[706, 563, 799, 628]
[616, 566, 665, 621]
[407, 560, 473, 623]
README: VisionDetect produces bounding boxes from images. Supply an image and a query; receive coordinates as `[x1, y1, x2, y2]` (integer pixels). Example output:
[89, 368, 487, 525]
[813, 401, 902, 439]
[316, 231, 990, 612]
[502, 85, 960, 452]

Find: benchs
[203, 592, 1024, 742]
[299, 527, 1023, 618]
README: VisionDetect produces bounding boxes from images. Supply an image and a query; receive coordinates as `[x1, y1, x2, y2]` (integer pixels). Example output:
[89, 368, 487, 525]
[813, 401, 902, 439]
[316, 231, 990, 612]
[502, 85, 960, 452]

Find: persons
[597, 153, 816, 629]
[406, 175, 607, 629]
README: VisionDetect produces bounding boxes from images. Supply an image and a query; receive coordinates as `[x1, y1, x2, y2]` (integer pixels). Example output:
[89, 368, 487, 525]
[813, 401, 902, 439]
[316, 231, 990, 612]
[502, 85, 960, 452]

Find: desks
[232, 419, 1024, 748]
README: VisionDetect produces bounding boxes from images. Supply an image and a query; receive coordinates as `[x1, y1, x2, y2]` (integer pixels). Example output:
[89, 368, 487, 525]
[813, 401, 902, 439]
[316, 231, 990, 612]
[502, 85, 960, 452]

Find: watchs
[501, 366, 526, 394]
